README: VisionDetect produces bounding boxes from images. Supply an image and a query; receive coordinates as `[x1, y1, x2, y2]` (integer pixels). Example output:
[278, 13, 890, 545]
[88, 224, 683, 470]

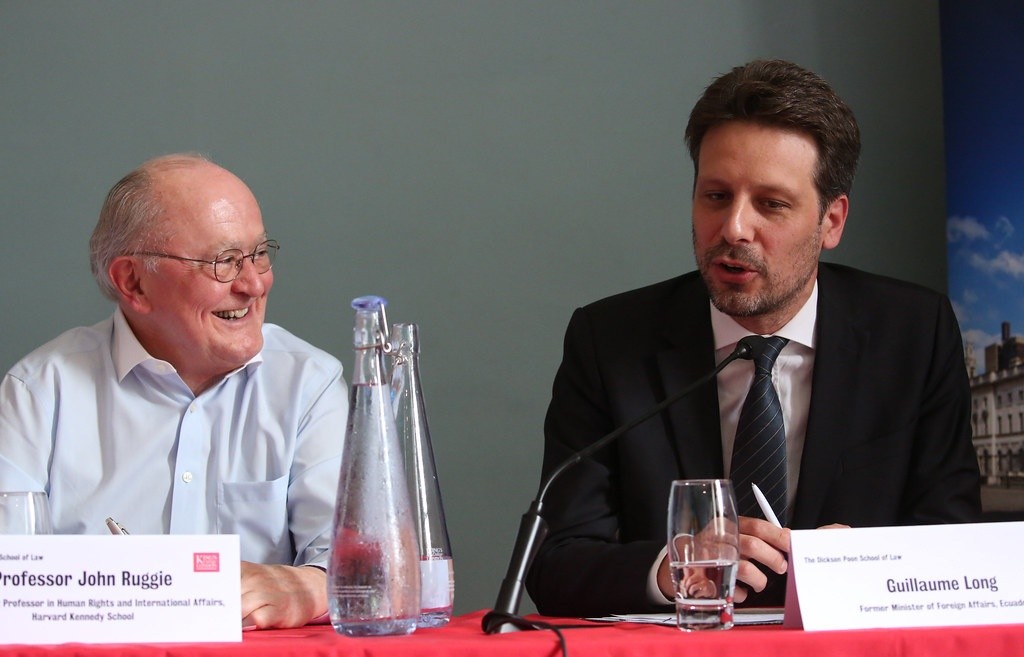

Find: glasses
[122, 238, 280, 283]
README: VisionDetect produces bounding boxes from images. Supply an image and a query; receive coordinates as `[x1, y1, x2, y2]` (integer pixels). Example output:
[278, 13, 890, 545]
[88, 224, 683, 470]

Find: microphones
[481, 335, 767, 638]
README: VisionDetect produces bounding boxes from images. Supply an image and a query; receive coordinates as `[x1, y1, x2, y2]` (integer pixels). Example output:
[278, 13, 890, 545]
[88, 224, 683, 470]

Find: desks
[0, 608, 1024, 657]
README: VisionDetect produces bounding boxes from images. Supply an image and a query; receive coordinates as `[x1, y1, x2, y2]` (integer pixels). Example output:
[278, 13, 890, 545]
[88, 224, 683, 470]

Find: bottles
[325, 293, 422, 638]
[388, 322, 455, 628]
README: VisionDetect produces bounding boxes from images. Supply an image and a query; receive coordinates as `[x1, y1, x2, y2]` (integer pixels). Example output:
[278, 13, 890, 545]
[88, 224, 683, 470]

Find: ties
[729, 336, 790, 608]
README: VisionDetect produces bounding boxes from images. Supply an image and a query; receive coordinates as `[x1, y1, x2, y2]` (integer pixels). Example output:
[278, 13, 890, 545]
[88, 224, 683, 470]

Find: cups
[666, 479, 739, 632]
[0, 490, 53, 538]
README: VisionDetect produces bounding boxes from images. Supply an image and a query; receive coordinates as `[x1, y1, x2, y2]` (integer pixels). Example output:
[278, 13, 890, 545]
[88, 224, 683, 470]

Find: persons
[1, 152, 354, 632]
[521, 56, 988, 618]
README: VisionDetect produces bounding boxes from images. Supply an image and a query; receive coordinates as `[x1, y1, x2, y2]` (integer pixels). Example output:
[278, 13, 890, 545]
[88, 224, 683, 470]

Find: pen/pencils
[106, 518, 130, 535]
[750, 481, 784, 529]
